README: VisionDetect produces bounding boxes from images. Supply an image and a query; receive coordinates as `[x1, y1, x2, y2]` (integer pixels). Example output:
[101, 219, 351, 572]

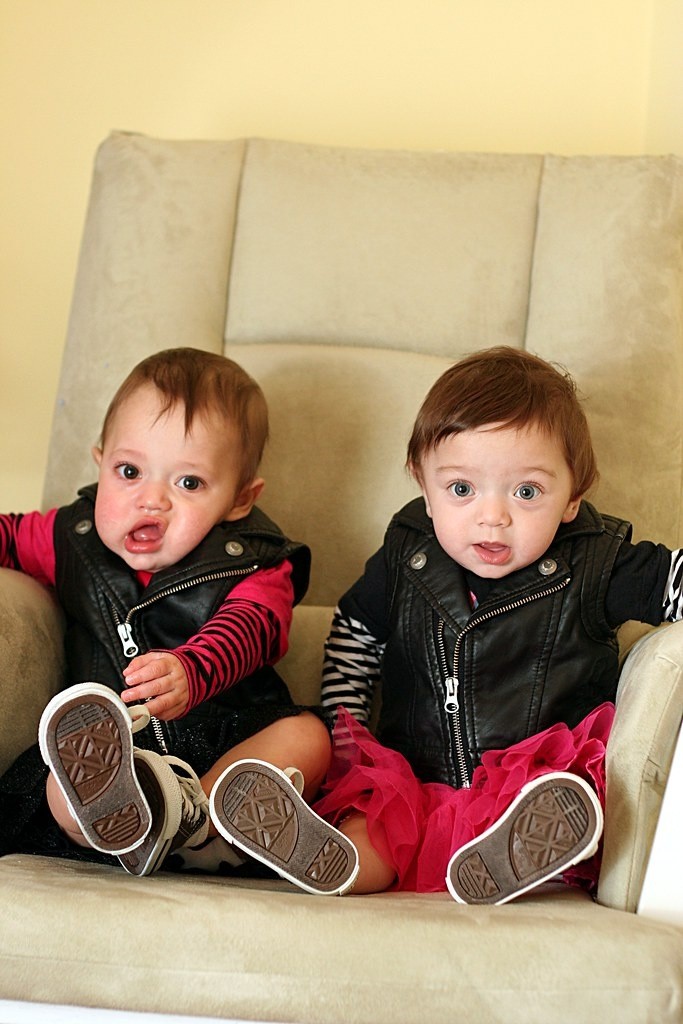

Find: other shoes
[115, 746, 211, 877]
[208, 759, 360, 897]
[443, 772, 604, 905]
[37, 682, 154, 856]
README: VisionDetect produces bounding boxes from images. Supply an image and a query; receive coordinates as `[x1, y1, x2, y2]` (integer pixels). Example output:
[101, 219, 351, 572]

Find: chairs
[0, 129, 683, 1024]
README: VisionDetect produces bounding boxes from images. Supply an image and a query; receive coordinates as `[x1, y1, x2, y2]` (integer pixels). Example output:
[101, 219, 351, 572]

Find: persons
[209, 346, 683, 906]
[0, 347, 330, 878]
